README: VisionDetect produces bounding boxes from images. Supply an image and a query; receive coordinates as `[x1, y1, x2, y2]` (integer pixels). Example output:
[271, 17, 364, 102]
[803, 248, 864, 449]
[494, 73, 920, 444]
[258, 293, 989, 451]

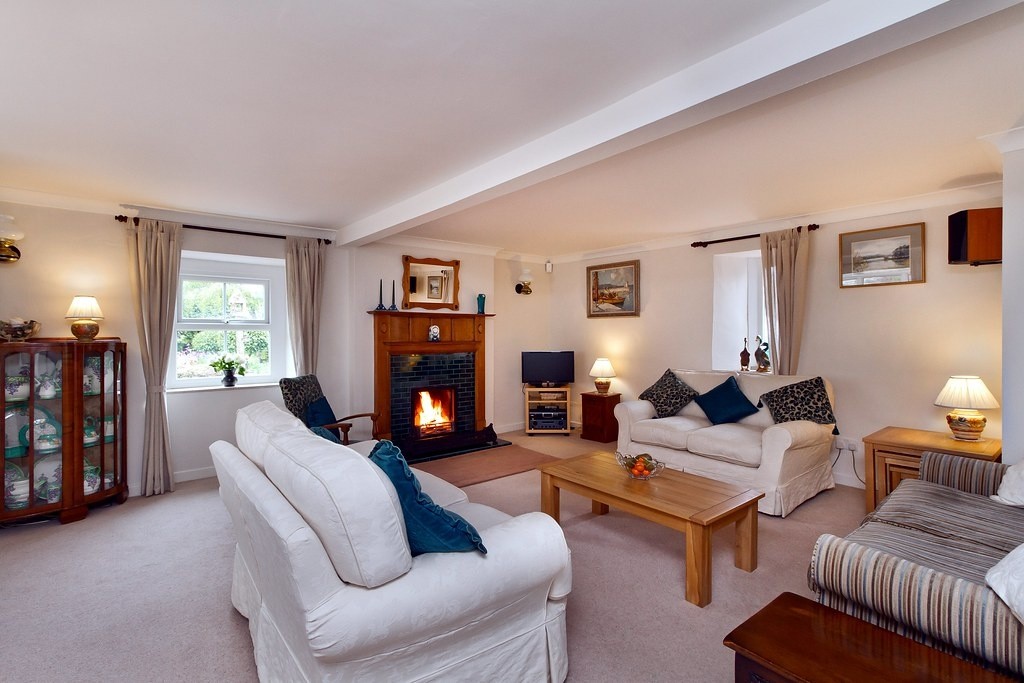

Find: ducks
[740, 338, 750, 371]
[754, 335, 770, 372]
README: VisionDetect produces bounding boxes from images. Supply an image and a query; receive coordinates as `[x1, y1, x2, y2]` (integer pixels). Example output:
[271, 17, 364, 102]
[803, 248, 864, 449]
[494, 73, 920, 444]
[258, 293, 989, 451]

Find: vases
[222, 370, 238, 387]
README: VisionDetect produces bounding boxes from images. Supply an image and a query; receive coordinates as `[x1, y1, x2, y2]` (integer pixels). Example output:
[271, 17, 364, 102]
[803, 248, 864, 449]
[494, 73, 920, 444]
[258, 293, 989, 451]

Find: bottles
[36, 434, 63, 454]
[54, 360, 63, 397]
[83, 426, 100, 447]
[477, 294, 486, 314]
[84, 466, 101, 494]
[83, 357, 121, 394]
[34, 374, 61, 398]
[83, 375, 91, 395]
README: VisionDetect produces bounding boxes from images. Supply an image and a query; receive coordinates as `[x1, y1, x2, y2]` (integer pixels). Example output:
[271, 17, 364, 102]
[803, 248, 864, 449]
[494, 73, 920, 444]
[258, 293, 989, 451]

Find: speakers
[947, 208, 1002, 264]
[409, 276, 417, 294]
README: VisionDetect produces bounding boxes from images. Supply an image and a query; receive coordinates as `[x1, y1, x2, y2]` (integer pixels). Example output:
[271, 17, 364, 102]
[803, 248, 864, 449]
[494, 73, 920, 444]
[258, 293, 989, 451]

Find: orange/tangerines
[632, 458, 651, 476]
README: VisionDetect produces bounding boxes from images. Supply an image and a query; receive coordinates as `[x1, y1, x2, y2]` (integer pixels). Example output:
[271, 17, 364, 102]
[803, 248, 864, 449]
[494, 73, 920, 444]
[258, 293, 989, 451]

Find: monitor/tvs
[522, 351, 574, 388]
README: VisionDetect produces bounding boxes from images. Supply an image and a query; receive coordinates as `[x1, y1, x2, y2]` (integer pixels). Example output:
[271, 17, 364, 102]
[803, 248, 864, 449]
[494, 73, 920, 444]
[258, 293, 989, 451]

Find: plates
[5, 404, 54, 446]
[19, 419, 63, 447]
[28, 452, 91, 500]
[5, 352, 55, 378]
[3, 459, 24, 484]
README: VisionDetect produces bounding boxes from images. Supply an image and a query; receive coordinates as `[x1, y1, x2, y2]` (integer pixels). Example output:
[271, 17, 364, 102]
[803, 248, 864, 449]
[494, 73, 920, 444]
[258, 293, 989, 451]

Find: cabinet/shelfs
[0, 333, 129, 526]
[524, 384, 571, 436]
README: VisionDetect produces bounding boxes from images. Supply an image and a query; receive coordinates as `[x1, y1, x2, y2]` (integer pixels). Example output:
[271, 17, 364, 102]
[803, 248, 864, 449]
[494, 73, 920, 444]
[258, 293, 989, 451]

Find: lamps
[934, 376, 1000, 442]
[513, 277, 533, 295]
[65, 296, 103, 341]
[589, 357, 616, 393]
[0, 214, 25, 262]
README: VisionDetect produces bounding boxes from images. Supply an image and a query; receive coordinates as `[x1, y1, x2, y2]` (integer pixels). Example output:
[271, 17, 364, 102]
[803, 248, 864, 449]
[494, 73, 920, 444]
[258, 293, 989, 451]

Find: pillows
[638, 368, 840, 434]
[367, 436, 489, 557]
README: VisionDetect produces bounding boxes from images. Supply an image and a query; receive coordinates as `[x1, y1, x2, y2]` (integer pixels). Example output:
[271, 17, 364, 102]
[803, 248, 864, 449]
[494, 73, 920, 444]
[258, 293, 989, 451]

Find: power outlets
[836, 437, 857, 451]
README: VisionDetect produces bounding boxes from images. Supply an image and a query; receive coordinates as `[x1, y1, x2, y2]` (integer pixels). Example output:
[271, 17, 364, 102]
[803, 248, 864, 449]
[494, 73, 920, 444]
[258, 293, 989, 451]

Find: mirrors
[401, 255, 460, 311]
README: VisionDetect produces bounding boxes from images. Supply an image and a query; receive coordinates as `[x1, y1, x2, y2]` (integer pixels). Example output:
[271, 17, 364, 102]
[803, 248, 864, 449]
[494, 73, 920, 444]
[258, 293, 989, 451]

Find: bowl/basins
[4, 375, 38, 401]
[0, 319, 41, 342]
[104, 472, 120, 489]
[5, 478, 43, 510]
[615, 452, 664, 481]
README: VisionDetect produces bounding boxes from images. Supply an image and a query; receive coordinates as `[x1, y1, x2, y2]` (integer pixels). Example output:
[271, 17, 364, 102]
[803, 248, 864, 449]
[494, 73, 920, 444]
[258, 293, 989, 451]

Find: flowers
[209, 352, 245, 376]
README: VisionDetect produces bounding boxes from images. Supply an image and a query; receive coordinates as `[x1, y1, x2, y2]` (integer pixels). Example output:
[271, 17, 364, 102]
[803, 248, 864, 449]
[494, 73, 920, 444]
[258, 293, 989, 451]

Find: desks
[535, 450, 766, 607]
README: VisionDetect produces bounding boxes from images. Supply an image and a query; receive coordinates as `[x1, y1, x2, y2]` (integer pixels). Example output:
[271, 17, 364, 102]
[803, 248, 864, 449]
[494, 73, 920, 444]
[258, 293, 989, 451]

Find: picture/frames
[427, 276, 442, 299]
[838, 222, 926, 288]
[586, 260, 640, 319]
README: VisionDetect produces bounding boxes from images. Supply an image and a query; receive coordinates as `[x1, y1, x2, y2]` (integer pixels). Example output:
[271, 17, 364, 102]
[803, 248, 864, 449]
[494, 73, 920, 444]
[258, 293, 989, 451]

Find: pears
[625, 453, 655, 475]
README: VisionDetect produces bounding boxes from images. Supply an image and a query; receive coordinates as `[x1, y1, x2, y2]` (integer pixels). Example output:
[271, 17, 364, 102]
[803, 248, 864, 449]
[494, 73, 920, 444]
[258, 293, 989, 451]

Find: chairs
[278, 371, 382, 441]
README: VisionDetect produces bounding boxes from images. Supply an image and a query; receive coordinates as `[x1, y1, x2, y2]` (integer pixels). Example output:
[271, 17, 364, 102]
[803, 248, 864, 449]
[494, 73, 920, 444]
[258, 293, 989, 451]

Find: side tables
[723, 592, 1017, 683]
[861, 426, 1001, 515]
[579, 391, 622, 444]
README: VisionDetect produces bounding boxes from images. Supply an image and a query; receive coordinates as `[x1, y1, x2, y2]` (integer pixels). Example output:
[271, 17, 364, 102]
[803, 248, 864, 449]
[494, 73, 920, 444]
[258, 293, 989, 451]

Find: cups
[98, 416, 119, 441]
[47, 483, 62, 504]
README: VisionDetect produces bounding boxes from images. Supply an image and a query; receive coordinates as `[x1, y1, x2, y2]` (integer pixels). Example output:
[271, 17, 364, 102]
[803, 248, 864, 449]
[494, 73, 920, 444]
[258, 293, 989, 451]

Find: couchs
[209, 373, 1024, 683]
